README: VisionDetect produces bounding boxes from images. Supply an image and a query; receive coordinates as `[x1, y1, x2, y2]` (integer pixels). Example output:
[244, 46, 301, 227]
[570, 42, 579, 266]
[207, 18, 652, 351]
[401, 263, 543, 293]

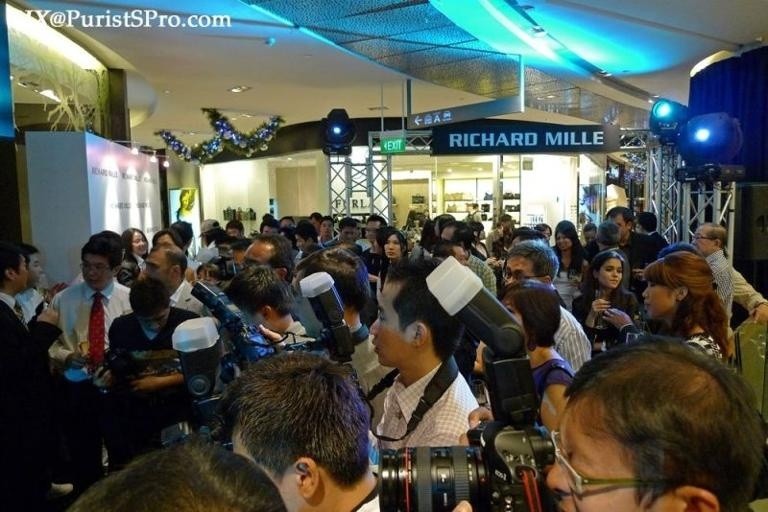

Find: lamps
[317, 106, 359, 158]
[671, 110, 746, 172]
[647, 94, 689, 153]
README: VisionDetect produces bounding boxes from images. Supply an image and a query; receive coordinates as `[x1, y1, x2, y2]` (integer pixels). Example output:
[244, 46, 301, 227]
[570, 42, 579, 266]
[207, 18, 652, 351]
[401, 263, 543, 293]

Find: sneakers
[44, 479, 74, 500]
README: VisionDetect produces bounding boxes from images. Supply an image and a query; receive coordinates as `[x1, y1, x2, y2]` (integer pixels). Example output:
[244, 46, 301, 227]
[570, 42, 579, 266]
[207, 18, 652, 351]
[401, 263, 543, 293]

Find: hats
[198, 219, 221, 238]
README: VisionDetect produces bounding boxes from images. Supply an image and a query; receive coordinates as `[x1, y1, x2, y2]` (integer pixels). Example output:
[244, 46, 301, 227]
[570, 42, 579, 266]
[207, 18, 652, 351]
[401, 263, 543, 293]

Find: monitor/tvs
[606, 155, 625, 189]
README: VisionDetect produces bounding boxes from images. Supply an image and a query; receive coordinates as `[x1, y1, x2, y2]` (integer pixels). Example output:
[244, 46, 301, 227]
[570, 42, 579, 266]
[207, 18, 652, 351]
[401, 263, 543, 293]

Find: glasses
[547, 427, 682, 500]
[500, 267, 544, 285]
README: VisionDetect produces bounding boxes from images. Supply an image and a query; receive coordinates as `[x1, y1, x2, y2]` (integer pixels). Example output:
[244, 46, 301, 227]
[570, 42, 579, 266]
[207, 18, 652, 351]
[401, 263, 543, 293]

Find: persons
[176, 190, 193, 222]
[1, 200, 767, 512]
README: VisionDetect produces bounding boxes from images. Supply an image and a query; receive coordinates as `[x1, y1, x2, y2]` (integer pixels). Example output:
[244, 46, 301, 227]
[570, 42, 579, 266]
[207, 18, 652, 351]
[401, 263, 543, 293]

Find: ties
[86, 291, 108, 374]
[11, 299, 29, 333]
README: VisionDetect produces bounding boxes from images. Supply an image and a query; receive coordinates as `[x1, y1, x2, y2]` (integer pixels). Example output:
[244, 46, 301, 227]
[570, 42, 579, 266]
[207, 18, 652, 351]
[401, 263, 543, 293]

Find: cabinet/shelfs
[432, 177, 440, 220]
[440, 176, 477, 223]
[475, 175, 521, 229]
[523, 200, 547, 228]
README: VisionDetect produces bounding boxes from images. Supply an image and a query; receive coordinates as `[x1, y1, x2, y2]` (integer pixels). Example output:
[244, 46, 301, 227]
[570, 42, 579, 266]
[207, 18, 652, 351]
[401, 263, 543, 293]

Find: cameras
[208, 252, 242, 280]
[378, 256, 555, 511]
[99, 347, 146, 385]
